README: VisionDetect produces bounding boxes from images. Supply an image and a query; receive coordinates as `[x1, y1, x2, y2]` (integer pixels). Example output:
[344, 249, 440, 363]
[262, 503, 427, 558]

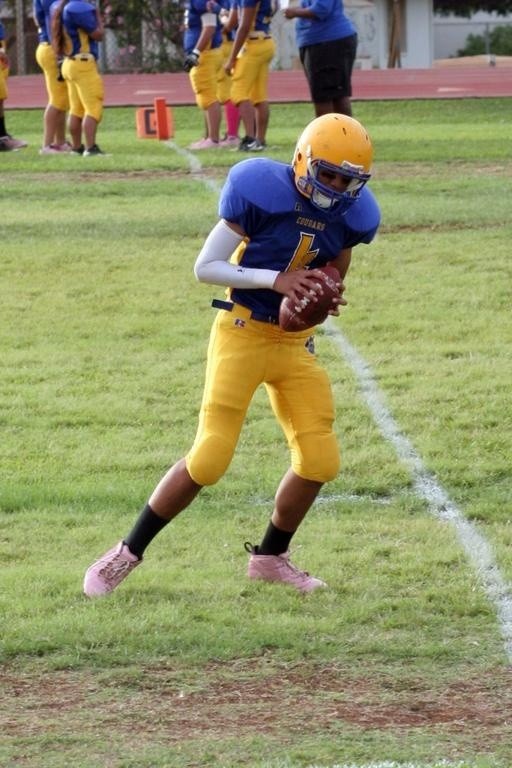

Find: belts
[250, 37, 271, 39]
[212, 299, 280, 324]
[70, 58, 88, 61]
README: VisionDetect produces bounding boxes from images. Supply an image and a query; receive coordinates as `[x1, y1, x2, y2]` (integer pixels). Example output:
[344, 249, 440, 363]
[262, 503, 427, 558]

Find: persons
[183, 0, 224, 151]
[32, 0, 72, 154]
[83, 112, 381, 600]
[0, 22, 28, 150]
[50, 0, 113, 157]
[283, 0, 358, 117]
[206, 0, 241, 146]
[223, 0, 279, 152]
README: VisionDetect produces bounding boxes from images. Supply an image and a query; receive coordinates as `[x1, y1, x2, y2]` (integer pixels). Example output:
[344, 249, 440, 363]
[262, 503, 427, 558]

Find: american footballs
[280, 267, 342, 332]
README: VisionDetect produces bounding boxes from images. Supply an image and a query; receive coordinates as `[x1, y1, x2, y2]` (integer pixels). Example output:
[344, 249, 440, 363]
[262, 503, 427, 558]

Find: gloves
[57, 59, 65, 80]
[184, 48, 201, 72]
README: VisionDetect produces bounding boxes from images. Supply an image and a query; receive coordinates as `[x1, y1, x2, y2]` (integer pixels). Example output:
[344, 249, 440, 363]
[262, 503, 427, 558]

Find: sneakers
[41, 141, 110, 157]
[244, 542, 325, 591]
[84, 541, 142, 596]
[0, 136, 27, 150]
[190, 135, 264, 151]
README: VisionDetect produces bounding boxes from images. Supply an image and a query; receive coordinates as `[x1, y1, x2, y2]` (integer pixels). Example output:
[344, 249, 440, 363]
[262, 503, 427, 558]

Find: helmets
[291, 113, 372, 217]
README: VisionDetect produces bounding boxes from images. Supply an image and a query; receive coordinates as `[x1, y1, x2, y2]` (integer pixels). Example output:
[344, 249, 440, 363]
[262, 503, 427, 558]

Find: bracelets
[192, 49, 200, 55]
